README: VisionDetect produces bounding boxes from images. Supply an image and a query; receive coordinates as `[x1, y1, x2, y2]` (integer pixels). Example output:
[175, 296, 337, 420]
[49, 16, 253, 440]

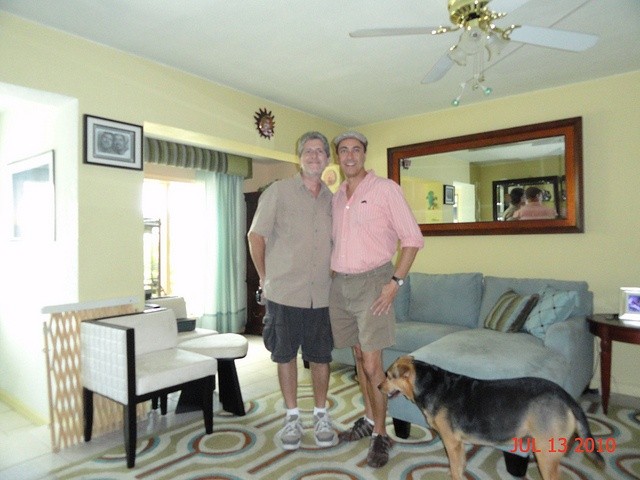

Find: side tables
[589, 310, 639, 416]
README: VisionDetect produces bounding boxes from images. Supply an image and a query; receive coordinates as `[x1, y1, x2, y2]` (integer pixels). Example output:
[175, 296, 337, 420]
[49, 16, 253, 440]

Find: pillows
[483, 287, 539, 334]
[523, 287, 579, 342]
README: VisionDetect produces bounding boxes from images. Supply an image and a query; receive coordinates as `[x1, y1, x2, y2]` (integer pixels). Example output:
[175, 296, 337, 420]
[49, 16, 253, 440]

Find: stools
[178, 331, 248, 416]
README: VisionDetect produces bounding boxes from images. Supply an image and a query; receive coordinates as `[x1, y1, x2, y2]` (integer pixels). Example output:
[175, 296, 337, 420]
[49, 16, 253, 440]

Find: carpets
[32, 367, 640, 480]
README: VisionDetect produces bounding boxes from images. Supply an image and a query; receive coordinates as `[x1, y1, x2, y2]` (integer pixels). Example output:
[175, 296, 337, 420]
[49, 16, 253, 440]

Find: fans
[346, 1, 600, 86]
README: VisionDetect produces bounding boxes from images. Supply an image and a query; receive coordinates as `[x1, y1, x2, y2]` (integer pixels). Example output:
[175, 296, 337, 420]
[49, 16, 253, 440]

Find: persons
[513, 187, 558, 220]
[503, 188, 525, 221]
[247, 131, 339, 450]
[328, 131, 424, 468]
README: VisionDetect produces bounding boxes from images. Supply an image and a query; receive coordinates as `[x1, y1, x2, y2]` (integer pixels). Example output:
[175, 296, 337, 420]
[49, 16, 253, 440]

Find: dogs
[378, 357, 606, 480]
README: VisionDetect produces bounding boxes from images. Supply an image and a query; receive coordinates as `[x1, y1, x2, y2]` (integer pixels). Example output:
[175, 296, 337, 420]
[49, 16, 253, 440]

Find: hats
[334, 131, 367, 153]
[526, 187, 544, 197]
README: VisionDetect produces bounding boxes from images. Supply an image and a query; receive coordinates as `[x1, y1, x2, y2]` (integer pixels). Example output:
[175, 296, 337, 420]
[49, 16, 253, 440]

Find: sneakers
[279, 414, 304, 450]
[338, 418, 374, 441]
[367, 434, 390, 468]
[313, 412, 338, 446]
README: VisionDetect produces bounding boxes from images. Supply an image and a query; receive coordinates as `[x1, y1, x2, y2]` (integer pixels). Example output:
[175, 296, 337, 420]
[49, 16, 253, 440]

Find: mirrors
[385, 116, 583, 234]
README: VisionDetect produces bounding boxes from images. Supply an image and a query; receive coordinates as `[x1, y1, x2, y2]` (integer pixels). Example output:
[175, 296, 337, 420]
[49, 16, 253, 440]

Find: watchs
[392, 276, 405, 286]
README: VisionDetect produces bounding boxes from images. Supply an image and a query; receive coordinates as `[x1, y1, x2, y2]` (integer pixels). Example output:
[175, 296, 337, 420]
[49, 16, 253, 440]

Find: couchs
[77, 309, 218, 469]
[147, 297, 218, 341]
[335, 273, 594, 478]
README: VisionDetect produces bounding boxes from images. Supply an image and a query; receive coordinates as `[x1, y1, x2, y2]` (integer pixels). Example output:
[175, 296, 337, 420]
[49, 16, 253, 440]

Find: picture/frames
[4, 148, 56, 246]
[442, 185, 456, 206]
[82, 114, 145, 170]
